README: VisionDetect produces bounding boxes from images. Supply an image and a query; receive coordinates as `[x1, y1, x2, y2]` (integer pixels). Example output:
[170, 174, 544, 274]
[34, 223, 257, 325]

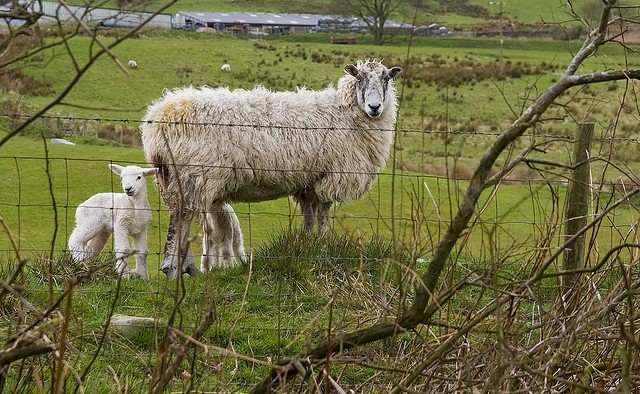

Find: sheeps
[129, 61, 137, 68]
[200, 202, 247, 274]
[68, 163, 160, 281]
[221, 64, 230, 71]
[139, 58, 402, 281]
